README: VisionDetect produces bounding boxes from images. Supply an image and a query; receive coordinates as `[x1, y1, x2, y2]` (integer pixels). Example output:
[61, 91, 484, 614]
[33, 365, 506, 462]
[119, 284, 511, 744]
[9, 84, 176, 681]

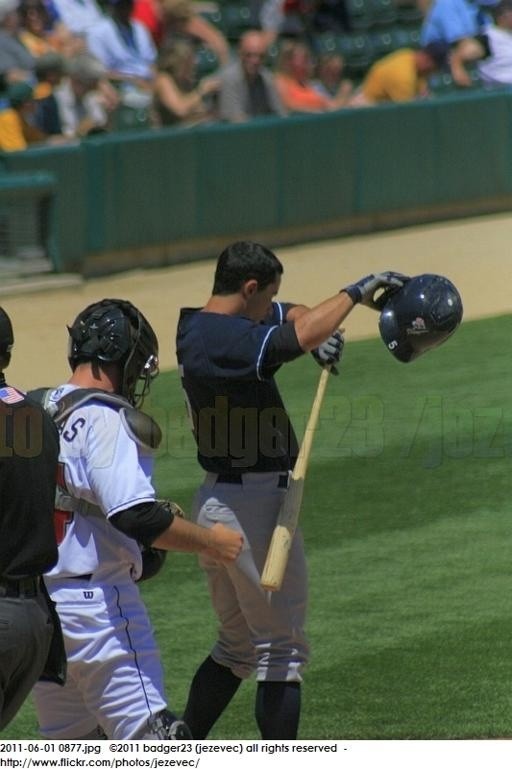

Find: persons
[174, 240, 408, 740]
[1, 0, 512, 151]
[1, 304, 60, 728]
[22, 296, 245, 738]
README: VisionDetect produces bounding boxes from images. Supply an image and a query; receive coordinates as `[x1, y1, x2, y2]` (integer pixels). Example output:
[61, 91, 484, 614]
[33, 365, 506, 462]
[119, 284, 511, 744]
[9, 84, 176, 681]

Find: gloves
[341, 271, 410, 310]
[310, 330, 344, 376]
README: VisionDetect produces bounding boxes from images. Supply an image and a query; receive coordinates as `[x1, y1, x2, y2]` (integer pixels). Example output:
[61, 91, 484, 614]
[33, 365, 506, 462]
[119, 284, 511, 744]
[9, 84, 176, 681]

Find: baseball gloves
[136, 502, 176, 583]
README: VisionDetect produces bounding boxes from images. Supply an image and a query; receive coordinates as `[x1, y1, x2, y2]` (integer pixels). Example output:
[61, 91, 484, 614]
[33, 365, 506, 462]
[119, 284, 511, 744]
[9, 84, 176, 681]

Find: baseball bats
[261, 326, 349, 591]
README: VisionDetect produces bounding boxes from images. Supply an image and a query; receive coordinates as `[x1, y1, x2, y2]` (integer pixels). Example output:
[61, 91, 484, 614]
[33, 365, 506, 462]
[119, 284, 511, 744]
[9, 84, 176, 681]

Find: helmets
[379, 274, 463, 363]
[66, 299, 161, 409]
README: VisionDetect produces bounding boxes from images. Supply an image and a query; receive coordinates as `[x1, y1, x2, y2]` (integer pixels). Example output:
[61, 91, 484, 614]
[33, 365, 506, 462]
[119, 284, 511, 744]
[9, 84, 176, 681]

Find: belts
[1, 573, 42, 598]
[216, 473, 288, 488]
